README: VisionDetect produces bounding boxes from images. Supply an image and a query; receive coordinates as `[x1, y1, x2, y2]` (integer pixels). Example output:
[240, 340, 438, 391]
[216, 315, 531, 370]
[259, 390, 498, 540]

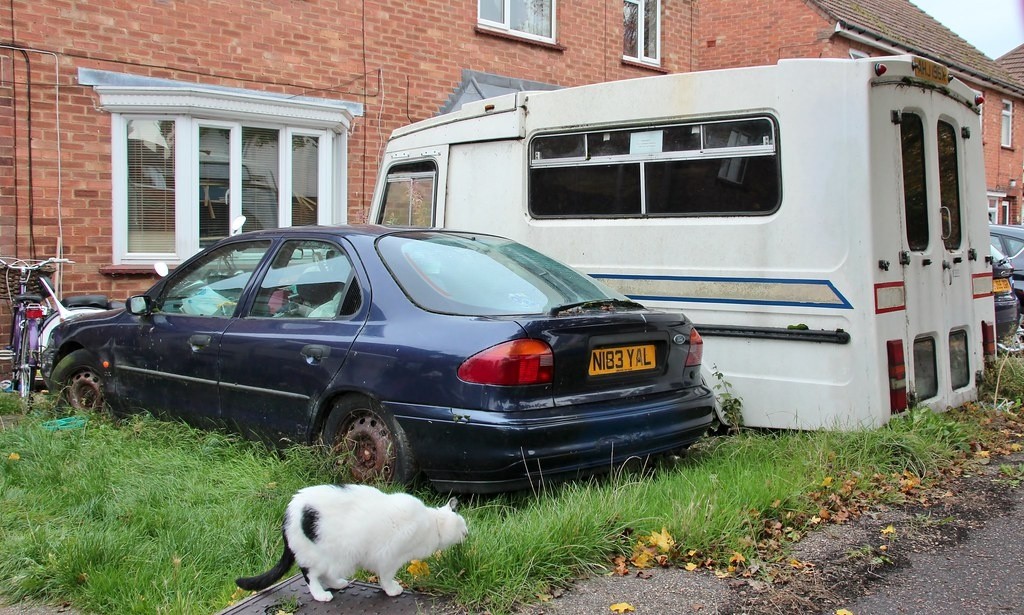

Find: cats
[234, 484, 468, 602]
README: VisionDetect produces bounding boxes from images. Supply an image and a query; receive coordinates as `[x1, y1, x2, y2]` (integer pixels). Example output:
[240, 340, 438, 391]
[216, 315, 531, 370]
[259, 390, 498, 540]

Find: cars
[43, 224, 717, 497]
[988, 224, 1024, 315]
[989, 243, 1019, 338]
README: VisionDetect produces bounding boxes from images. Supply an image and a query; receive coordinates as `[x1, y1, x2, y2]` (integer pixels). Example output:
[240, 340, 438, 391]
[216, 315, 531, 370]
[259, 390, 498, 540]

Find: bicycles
[1, 257, 75, 413]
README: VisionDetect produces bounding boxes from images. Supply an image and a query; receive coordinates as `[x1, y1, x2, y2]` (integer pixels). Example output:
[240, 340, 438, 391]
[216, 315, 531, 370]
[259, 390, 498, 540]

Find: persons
[285, 273, 342, 318]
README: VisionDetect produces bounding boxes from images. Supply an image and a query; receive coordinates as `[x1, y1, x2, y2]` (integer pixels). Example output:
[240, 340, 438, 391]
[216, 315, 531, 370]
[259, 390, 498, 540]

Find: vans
[364, 54, 1002, 436]
